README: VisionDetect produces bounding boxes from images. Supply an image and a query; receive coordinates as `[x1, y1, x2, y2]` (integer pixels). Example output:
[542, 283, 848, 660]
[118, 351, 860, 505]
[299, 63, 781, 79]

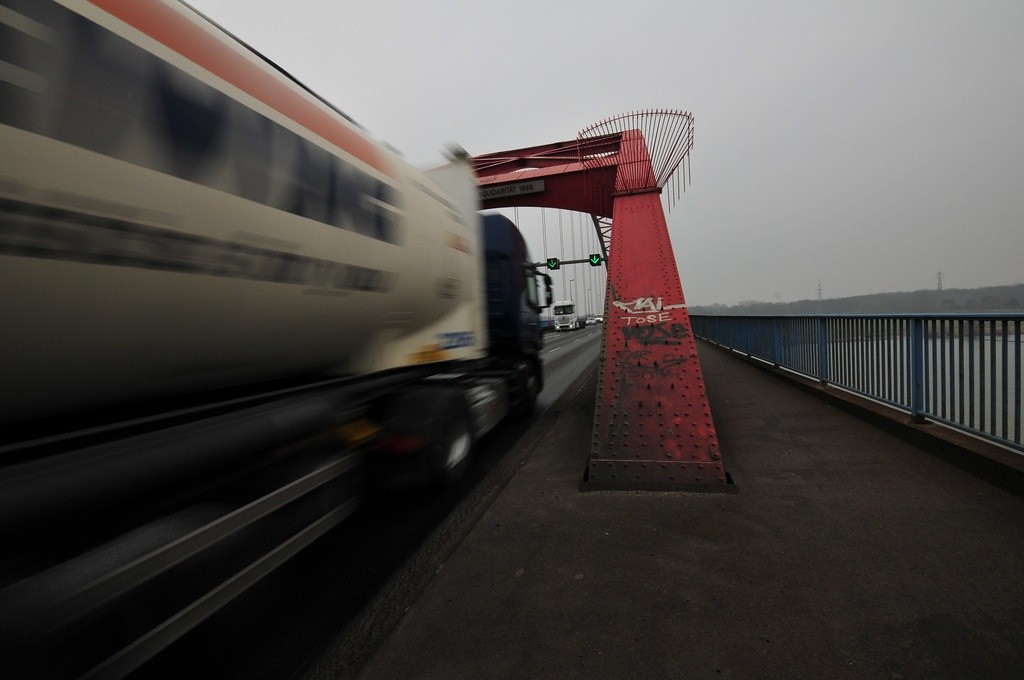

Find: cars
[585, 317, 596, 326]
[594, 314, 603, 323]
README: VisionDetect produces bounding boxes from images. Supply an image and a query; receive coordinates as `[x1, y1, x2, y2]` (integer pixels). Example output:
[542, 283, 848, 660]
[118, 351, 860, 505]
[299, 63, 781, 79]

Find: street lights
[570, 279, 574, 301]
[587, 288, 591, 318]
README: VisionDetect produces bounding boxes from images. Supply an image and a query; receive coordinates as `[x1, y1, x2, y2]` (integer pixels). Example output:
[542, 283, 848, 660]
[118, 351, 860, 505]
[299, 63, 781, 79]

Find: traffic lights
[546, 258, 559, 270]
[588, 254, 600, 265]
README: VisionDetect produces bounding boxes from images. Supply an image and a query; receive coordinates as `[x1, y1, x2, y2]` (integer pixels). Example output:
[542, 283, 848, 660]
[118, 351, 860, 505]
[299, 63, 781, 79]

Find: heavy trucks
[552, 300, 586, 331]
[0, 3, 554, 680]
